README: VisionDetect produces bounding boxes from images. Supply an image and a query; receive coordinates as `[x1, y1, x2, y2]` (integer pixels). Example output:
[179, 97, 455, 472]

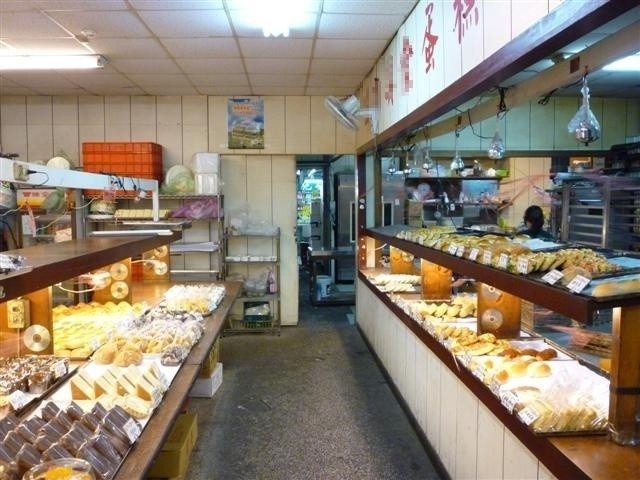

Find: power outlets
[109, 178, 118, 189]
[14, 162, 31, 181]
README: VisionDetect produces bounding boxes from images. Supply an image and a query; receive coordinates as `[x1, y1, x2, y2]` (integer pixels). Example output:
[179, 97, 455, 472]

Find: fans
[324, 95, 379, 135]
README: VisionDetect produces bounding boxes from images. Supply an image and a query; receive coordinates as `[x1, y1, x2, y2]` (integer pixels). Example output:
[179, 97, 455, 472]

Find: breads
[420, 300, 608, 435]
[53, 332, 192, 367]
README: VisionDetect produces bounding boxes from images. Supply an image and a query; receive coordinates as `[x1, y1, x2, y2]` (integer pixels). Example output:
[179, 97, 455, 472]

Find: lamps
[0, 54, 110, 71]
[388, 77, 601, 177]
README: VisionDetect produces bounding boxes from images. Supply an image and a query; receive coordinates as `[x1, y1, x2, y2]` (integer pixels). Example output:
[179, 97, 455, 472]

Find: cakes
[0, 354, 69, 420]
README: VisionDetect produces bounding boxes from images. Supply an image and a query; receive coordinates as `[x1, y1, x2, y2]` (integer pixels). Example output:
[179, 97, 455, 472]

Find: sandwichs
[0, 363, 167, 480]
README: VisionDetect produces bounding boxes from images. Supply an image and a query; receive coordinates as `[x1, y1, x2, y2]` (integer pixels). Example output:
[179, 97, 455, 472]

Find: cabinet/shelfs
[17, 190, 281, 338]
[561, 174, 640, 323]
[356, 227, 640, 479]
[0, 158, 243, 480]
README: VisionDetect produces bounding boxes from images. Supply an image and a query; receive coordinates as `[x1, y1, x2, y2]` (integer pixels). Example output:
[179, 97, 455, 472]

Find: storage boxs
[146, 413, 198, 480]
[82, 141, 163, 196]
[188, 362, 223, 397]
[199, 334, 220, 378]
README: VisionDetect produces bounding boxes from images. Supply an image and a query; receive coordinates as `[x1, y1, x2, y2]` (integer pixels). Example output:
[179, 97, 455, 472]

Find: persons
[514, 205, 556, 242]
